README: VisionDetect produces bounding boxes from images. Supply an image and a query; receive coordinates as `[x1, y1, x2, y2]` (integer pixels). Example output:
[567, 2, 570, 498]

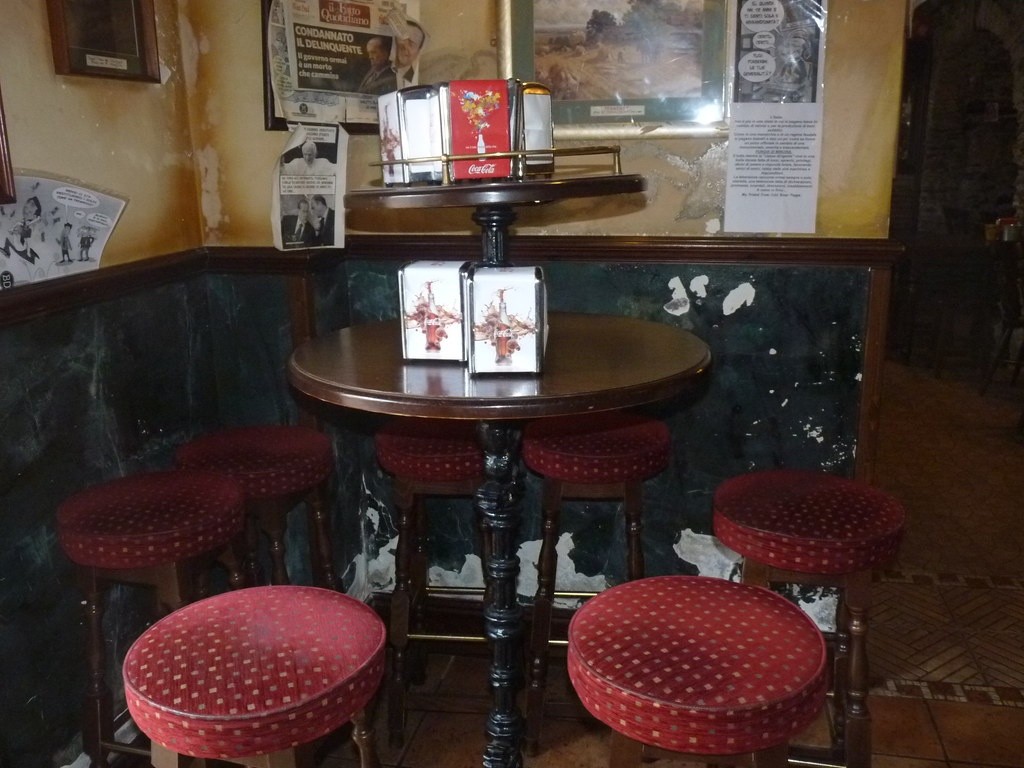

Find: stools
[56, 426, 386, 768]
[981, 268, 1024, 396]
[376, 411, 905, 768]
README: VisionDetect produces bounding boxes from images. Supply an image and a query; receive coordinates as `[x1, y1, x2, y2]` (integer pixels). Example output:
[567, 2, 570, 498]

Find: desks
[286, 312, 712, 768]
[897, 239, 1024, 365]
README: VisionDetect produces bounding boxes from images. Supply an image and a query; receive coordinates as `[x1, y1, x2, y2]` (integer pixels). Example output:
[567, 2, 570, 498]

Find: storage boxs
[377, 80, 511, 184]
[397, 258, 549, 374]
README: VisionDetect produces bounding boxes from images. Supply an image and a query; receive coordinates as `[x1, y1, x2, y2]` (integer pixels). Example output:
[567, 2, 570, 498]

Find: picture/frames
[495, 0, 737, 140]
[261, 0, 381, 135]
[46, 0, 161, 84]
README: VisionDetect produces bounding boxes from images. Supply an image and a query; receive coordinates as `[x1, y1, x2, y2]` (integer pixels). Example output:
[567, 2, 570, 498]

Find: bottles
[427, 294, 440, 350]
[497, 303, 511, 358]
